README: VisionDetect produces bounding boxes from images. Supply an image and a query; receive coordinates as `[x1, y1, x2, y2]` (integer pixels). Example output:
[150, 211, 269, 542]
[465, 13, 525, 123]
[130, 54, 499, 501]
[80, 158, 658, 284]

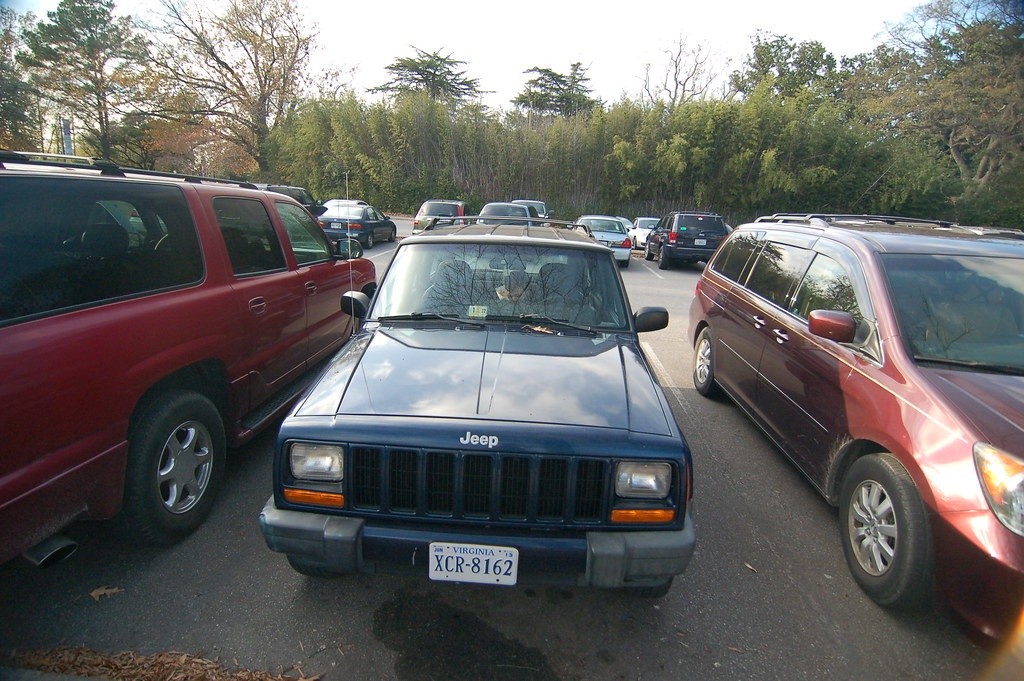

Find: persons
[492, 270, 564, 319]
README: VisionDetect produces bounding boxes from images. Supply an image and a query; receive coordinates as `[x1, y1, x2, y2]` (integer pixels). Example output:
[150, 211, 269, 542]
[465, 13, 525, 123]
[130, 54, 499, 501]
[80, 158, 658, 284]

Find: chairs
[150, 225, 202, 281]
[73, 222, 134, 283]
[422, 261, 480, 313]
[537, 262, 583, 305]
[926, 276, 1018, 342]
[607, 223, 616, 230]
[222, 225, 266, 275]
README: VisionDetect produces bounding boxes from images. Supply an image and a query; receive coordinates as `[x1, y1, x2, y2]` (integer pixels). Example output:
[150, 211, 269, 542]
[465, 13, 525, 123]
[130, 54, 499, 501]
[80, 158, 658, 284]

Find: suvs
[255, 212, 699, 601]
[685, 212, 1024, 657]
[0, 148, 377, 571]
[476, 201, 542, 228]
[213, 182, 328, 244]
[507, 199, 556, 227]
[642, 209, 729, 271]
[410, 198, 471, 235]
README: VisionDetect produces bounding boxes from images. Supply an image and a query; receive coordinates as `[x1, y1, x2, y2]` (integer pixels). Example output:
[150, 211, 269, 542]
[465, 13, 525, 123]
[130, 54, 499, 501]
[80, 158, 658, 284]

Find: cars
[614, 215, 661, 251]
[128, 207, 169, 236]
[566, 213, 632, 268]
[317, 198, 397, 250]
